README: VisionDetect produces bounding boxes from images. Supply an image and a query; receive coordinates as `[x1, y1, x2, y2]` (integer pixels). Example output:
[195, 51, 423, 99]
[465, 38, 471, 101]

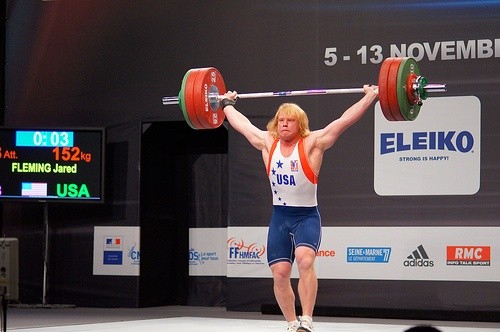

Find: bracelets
[221, 97, 240, 110]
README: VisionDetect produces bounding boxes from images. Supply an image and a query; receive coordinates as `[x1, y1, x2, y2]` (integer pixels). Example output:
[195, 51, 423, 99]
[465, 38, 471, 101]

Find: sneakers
[296, 316, 313, 332]
[286, 320, 300, 332]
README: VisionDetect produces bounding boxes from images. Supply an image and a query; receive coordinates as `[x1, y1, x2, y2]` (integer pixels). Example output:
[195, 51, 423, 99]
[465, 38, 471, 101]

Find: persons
[220, 84, 381, 332]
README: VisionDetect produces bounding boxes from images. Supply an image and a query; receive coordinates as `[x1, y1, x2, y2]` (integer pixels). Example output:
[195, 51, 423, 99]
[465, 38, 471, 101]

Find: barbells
[161, 56, 447, 130]
